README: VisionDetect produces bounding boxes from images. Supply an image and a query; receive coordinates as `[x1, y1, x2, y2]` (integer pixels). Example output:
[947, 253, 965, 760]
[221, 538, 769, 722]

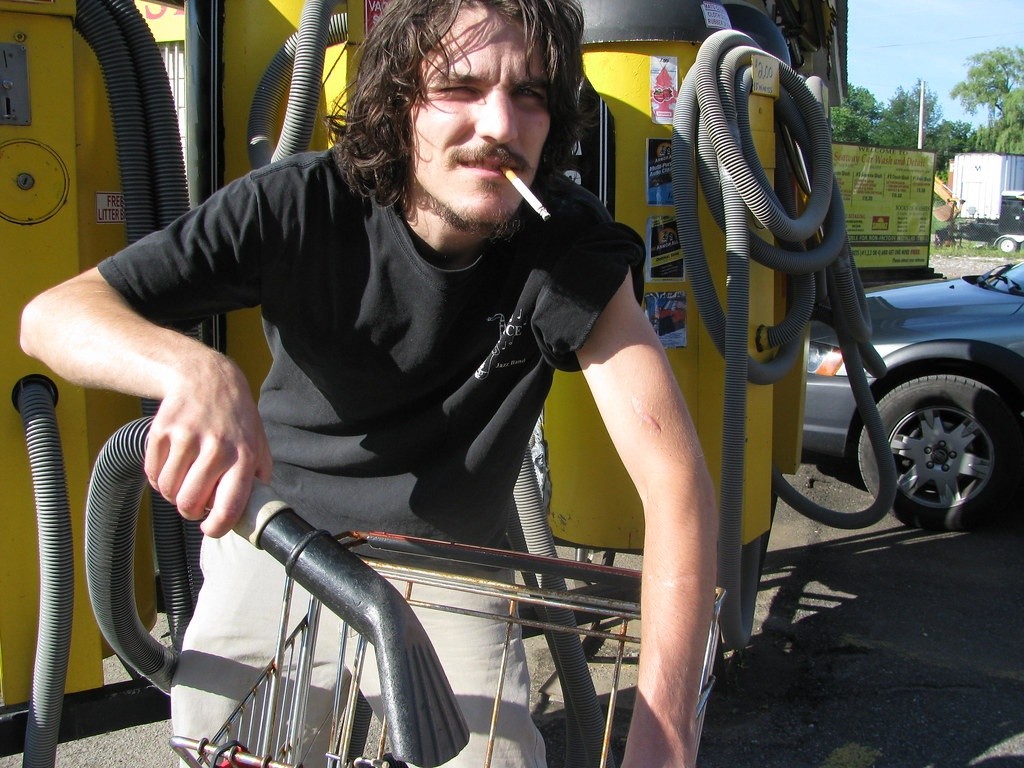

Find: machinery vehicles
[932, 149, 1024, 254]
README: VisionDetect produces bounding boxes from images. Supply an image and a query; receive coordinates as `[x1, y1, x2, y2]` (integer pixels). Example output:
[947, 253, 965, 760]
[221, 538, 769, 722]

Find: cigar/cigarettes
[498, 165, 550, 220]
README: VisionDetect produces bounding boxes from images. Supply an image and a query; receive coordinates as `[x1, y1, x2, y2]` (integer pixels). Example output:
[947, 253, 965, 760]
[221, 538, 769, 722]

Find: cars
[799, 257, 1024, 532]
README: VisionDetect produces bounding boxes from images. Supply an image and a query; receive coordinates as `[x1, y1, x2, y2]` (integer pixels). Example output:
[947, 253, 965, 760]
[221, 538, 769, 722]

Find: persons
[19, 0, 718, 768]
